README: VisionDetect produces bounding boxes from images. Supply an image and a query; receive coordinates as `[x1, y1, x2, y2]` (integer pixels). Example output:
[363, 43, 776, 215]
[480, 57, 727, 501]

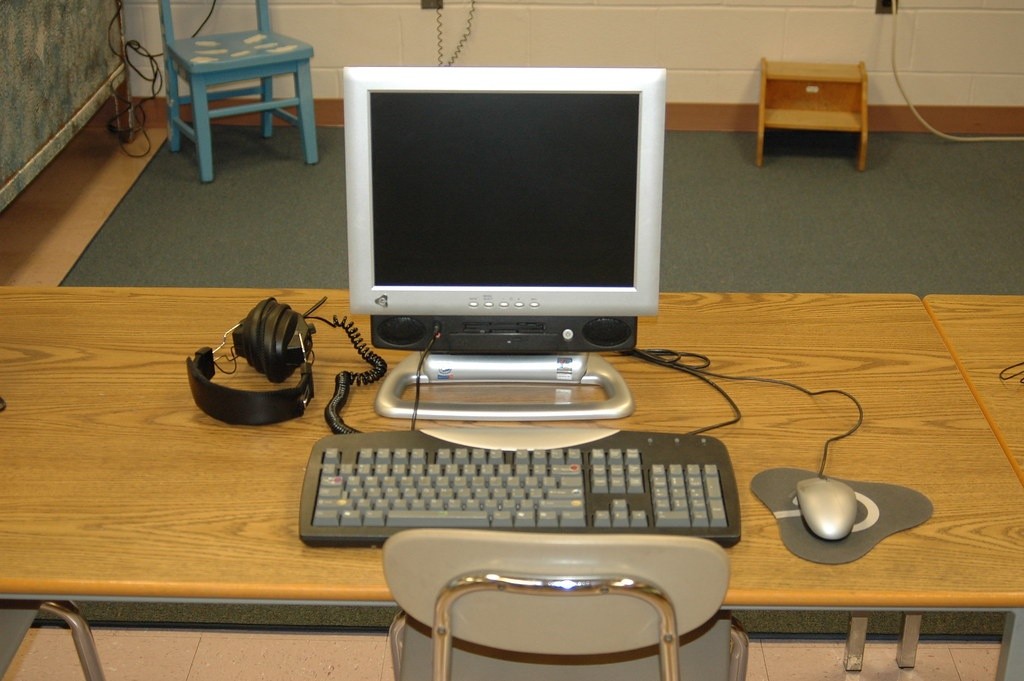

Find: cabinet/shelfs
[756, 59, 868, 169]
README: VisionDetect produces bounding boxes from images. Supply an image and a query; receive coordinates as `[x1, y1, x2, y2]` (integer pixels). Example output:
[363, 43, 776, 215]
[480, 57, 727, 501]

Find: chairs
[381, 528, 749, 680]
[159, 0, 318, 182]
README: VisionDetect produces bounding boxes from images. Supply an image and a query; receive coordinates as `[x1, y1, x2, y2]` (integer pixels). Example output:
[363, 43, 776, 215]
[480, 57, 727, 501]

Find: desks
[895, 296, 1024, 669]
[1, 286, 1024, 681]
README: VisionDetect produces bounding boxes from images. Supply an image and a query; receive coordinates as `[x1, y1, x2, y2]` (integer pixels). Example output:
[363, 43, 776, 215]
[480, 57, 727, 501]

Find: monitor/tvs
[341, 68, 664, 419]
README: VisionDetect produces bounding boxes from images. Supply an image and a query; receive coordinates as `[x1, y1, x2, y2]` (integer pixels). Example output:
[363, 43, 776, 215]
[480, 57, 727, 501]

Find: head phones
[185, 294, 318, 426]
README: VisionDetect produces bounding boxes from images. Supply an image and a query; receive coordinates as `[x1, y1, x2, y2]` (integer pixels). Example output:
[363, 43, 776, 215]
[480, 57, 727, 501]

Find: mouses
[796, 475, 858, 541]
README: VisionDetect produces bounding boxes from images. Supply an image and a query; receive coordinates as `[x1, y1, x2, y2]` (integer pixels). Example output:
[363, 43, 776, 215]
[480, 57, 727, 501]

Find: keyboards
[297, 421, 742, 549]
